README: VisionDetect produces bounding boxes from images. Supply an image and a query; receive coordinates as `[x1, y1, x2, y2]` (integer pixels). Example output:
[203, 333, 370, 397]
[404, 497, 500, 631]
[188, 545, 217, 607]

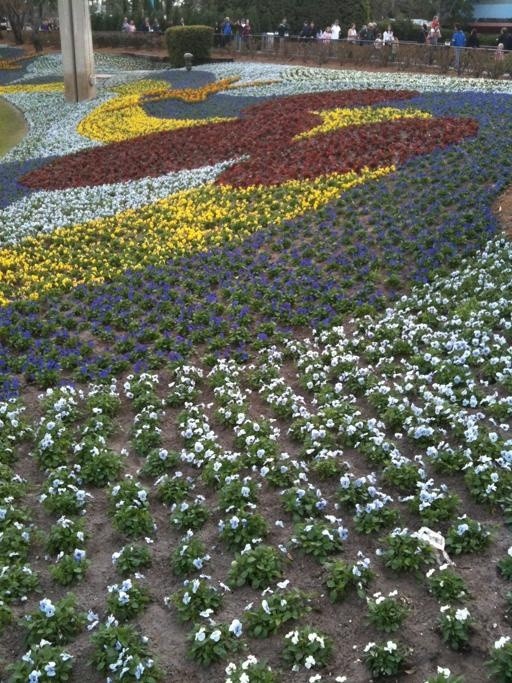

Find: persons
[494, 27, 512, 68]
[431, 14, 439, 28]
[449, 22, 467, 69]
[23, 17, 59, 31]
[495, 42, 506, 63]
[121, 15, 401, 65]
[464, 27, 481, 68]
[427, 22, 441, 66]
